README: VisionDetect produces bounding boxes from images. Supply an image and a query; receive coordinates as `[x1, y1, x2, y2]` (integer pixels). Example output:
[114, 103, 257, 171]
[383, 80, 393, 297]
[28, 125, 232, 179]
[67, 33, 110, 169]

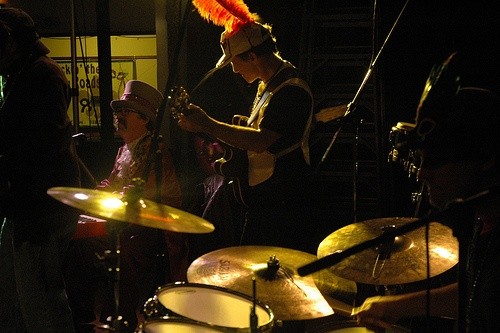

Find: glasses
[113, 109, 142, 116]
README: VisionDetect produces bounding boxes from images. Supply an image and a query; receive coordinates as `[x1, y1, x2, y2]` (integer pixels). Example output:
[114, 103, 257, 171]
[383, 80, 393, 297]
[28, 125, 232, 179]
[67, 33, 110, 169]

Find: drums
[137, 282, 417, 333]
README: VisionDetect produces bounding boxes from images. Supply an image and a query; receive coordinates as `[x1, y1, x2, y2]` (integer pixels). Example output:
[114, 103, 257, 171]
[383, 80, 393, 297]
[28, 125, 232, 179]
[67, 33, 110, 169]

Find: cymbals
[45, 186, 215, 235]
[187, 244, 360, 320]
[316, 216, 466, 286]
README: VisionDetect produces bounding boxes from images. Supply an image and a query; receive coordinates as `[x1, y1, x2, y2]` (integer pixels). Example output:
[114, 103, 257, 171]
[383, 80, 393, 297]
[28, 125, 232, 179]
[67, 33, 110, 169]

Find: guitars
[167, 84, 253, 208]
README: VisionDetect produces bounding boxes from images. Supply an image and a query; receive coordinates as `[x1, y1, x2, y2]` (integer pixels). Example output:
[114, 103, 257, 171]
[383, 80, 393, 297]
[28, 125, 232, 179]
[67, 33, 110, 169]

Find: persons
[70, 81, 183, 333]
[178, 0, 322, 255]
[0, 8, 81, 333]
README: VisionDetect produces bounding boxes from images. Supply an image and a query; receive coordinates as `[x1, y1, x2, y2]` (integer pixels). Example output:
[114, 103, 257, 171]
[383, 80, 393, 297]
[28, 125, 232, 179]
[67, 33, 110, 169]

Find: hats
[110, 81, 164, 122]
[192, 0, 271, 69]
[0, 8, 50, 54]
